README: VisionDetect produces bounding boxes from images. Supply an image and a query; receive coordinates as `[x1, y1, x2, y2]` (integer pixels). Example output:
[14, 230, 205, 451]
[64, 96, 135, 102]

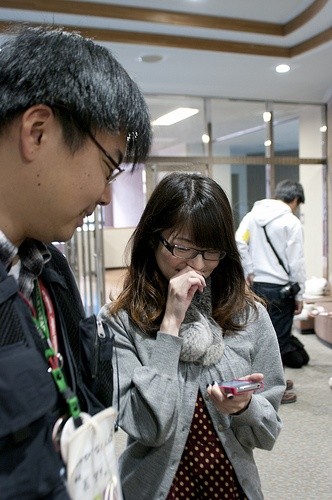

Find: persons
[0, 25, 154, 500]
[236, 180, 307, 403]
[97, 172, 286, 500]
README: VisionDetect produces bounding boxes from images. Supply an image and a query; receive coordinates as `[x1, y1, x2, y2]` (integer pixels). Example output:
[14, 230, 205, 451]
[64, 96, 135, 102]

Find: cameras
[280, 281, 301, 299]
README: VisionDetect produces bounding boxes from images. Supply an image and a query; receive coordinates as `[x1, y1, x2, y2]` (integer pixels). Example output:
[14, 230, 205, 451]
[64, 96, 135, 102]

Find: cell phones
[217, 378, 264, 395]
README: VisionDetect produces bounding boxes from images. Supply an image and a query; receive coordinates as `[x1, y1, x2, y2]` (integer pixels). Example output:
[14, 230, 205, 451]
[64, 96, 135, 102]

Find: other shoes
[281, 392, 297, 404]
[286, 380, 293, 388]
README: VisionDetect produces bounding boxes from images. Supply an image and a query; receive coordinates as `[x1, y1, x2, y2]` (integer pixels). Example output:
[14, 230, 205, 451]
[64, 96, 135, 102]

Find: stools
[293, 295, 332, 349]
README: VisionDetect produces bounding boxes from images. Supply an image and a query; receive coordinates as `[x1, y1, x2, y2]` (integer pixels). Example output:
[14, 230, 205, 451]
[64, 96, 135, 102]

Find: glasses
[74, 117, 125, 184]
[158, 236, 227, 262]
